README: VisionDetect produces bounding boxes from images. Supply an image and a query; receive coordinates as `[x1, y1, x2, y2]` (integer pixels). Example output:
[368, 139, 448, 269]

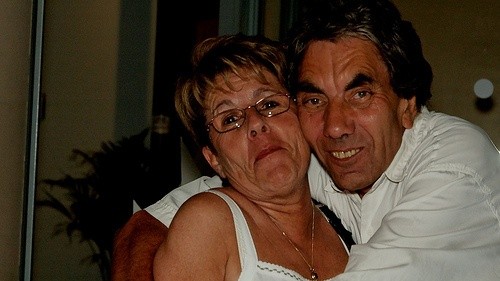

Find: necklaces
[267, 198, 319, 279]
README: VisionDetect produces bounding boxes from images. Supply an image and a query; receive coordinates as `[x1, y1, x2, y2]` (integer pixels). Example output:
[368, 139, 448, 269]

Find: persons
[112, 0, 500, 281]
[151, 33, 356, 281]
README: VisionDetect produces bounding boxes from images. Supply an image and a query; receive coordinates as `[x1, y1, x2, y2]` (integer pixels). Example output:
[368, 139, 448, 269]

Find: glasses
[206, 88, 296, 133]
